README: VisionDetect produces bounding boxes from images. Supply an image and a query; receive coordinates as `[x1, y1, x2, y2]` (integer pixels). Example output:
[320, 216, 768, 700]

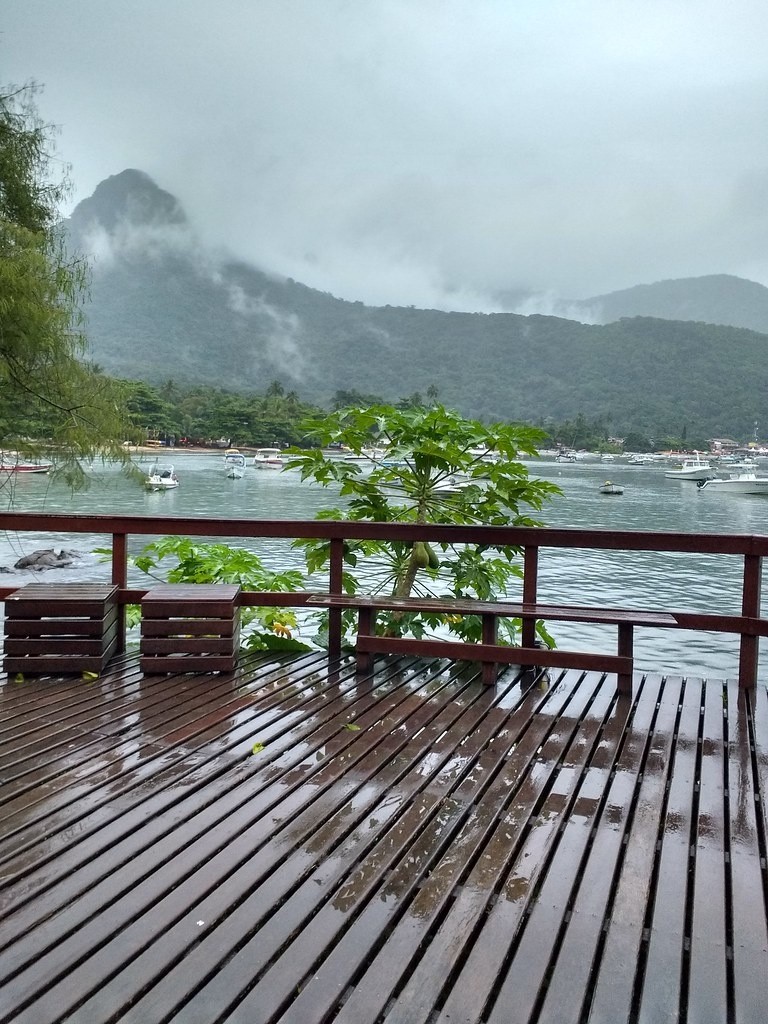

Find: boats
[598, 479, 625, 495]
[627, 457, 646, 465]
[0, 463, 53, 474]
[222, 449, 246, 479]
[254, 448, 284, 470]
[344, 444, 505, 494]
[602, 454, 615, 462]
[555, 453, 577, 464]
[144, 464, 180, 492]
[697, 470, 768, 495]
[664, 459, 718, 480]
[715, 453, 768, 470]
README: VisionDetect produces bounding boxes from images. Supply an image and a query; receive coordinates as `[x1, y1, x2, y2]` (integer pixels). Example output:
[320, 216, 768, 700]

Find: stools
[2, 582, 120, 678]
[138, 584, 242, 677]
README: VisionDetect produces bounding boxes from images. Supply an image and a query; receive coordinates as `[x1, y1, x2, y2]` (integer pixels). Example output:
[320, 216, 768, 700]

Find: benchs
[304, 591, 678, 696]
